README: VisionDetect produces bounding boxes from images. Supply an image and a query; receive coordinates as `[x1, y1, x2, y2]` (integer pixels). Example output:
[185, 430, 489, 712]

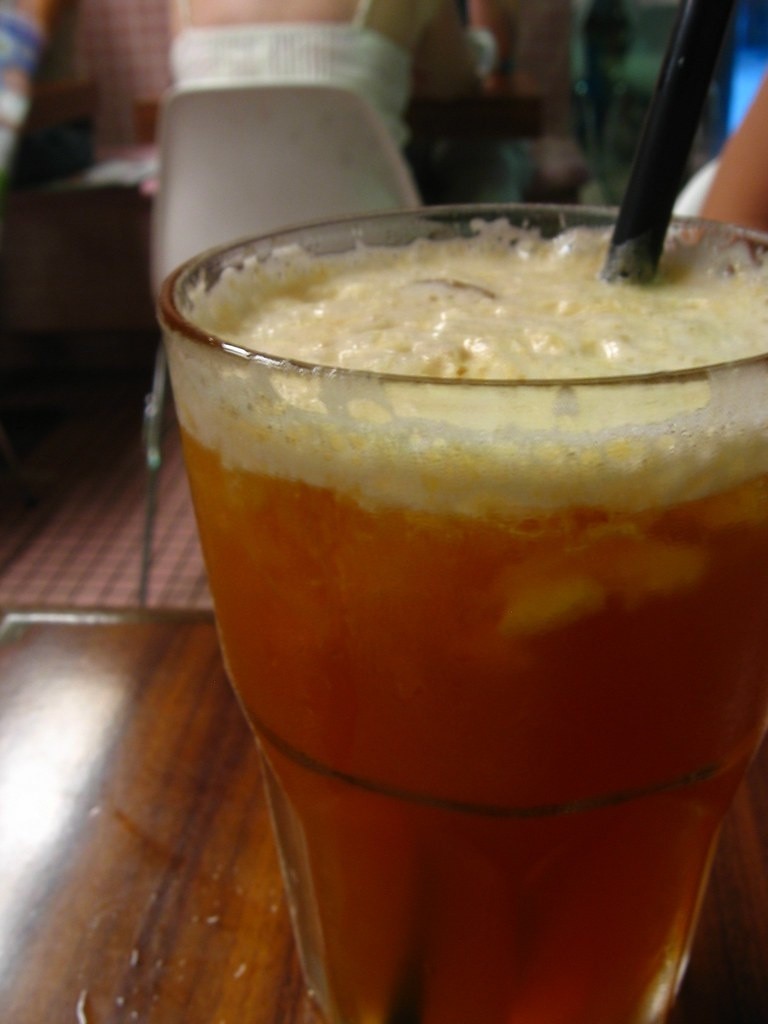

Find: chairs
[136, 82, 422, 604]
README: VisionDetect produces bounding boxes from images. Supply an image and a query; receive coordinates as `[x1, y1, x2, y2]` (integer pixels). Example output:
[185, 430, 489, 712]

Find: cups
[158, 200, 768, 1024]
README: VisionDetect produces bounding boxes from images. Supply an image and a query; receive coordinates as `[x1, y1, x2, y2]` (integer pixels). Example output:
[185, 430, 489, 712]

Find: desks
[138, 67, 546, 141]
[0, 600, 768, 1024]
